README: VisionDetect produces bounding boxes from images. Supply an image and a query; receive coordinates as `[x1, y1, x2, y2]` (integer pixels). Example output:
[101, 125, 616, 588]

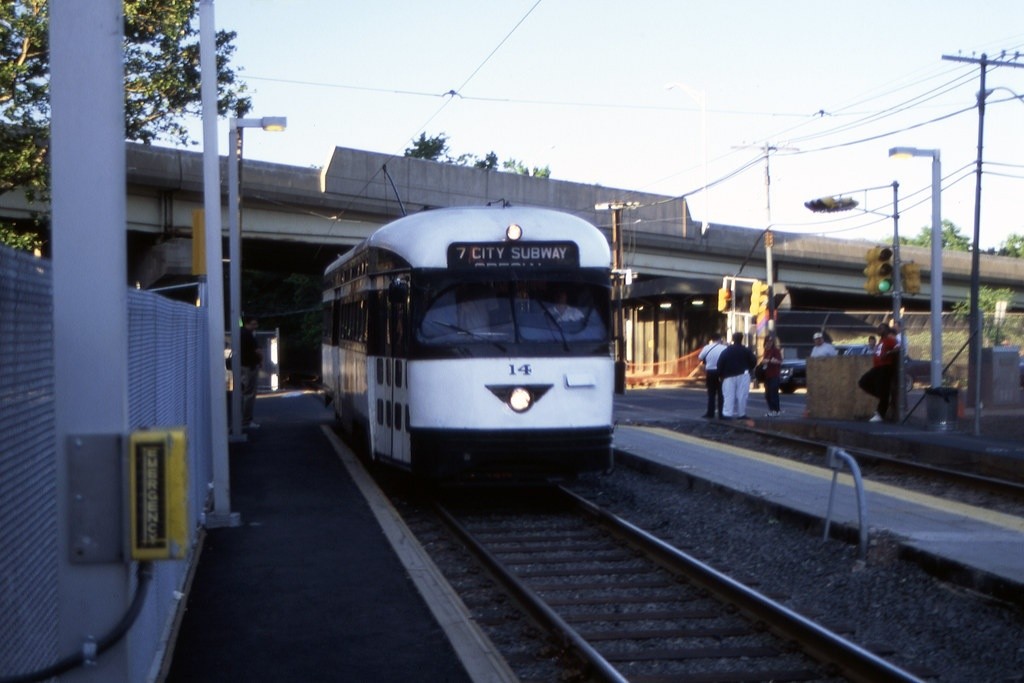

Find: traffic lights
[718, 289, 732, 311]
[750, 281, 769, 315]
[803, 197, 835, 213]
[862, 247, 893, 295]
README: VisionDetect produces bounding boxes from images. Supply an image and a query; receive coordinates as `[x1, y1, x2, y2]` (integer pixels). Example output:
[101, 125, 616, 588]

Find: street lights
[595, 201, 640, 395]
[228, 116, 286, 442]
[888, 146, 942, 387]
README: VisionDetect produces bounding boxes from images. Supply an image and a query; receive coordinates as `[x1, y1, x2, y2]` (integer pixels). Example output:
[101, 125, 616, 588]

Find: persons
[240, 317, 262, 427]
[698, 333, 724, 418]
[760, 335, 782, 415]
[858, 321, 908, 422]
[541, 289, 585, 323]
[422, 282, 491, 336]
[717, 333, 757, 422]
[810, 333, 836, 357]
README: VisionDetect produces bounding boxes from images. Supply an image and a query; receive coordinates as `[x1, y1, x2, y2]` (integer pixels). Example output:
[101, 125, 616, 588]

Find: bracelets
[769, 359, 771, 363]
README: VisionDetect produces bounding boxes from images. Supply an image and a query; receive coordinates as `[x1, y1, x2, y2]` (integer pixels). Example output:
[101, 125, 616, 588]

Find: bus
[322, 202, 629, 490]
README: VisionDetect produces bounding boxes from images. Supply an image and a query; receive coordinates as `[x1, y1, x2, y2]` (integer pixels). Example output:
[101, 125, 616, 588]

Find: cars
[779, 345, 930, 394]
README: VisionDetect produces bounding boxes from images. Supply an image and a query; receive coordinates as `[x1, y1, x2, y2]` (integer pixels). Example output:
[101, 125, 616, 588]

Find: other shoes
[701, 414, 713, 418]
[720, 416, 732, 421]
[869, 411, 884, 422]
[767, 410, 780, 417]
[737, 415, 750, 420]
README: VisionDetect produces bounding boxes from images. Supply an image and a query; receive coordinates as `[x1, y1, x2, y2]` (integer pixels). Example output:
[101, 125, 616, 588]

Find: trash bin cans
[926, 387, 958, 431]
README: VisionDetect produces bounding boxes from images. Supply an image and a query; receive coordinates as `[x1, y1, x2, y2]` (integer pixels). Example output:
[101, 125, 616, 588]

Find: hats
[813, 332, 823, 339]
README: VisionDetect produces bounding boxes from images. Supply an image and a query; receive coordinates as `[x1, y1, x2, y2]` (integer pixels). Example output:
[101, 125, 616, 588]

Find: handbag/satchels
[755, 364, 768, 381]
